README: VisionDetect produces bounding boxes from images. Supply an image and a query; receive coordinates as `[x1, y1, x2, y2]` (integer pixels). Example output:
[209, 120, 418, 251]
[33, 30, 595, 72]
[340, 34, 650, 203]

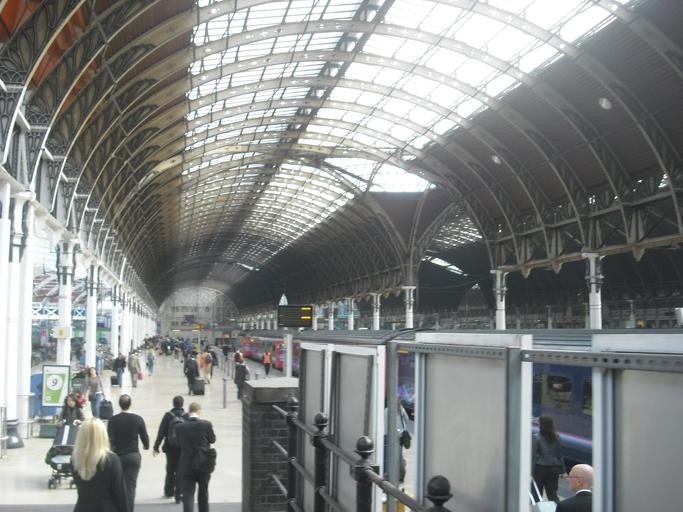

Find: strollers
[44, 423, 82, 489]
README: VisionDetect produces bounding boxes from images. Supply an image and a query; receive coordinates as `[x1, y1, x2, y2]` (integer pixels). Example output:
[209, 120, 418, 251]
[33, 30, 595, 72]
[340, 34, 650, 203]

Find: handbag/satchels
[399, 430, 412, 449]
[529, 477, 558, 512]
[184, 368, 190, 376]
[137, 372, 144, 381]
[190, 447, 216, 475]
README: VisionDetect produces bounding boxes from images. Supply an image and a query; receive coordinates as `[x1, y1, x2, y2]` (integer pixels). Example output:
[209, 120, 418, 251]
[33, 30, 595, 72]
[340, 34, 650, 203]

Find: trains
[240, 328, 683, 474]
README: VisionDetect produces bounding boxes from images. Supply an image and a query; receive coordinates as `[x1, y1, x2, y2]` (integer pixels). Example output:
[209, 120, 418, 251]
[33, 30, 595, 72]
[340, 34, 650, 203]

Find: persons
[383, 388, 410, 485]
[82, 366, 107, 419]
[105, 393, 149, 512]
[69, 416, 130, 512]
[532, 414, 566, 506]
[553, 462, 593, 511]
[152, 395, 189, 504]
[112, 333, 274, 400]
[30, 336, 111, 368]
[55, 393, 88, 480]
[172, 401, 216, 512]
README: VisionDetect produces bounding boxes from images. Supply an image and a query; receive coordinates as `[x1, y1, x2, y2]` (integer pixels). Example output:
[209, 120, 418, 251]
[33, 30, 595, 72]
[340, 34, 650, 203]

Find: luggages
[111, 375, 118, 386]
[99, 399, 113, 420]
[192, 376, 205, 395]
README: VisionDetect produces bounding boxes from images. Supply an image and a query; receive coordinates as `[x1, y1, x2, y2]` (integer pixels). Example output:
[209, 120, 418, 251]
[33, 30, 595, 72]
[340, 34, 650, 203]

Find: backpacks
[165, 411, 186, 451]
